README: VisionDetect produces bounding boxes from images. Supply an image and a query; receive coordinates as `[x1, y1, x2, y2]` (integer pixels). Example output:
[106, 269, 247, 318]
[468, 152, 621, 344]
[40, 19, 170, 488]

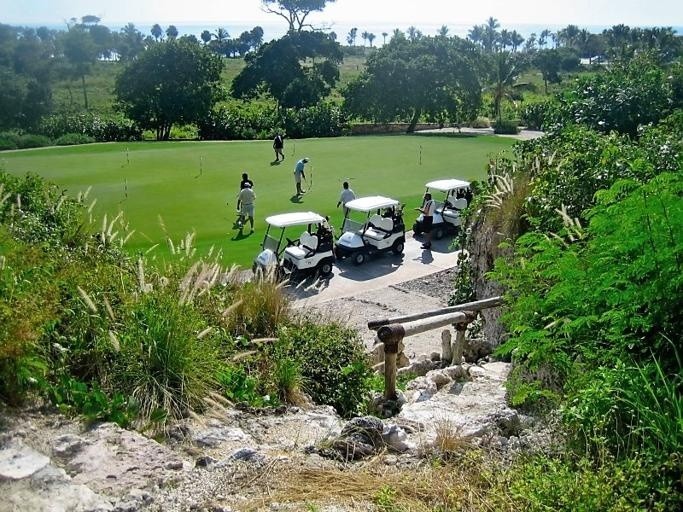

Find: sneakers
[420, 242, 431, 249]
[239, 224, 254, 232]
[275, 155, 284, 162]
[298, 190, 306, 195]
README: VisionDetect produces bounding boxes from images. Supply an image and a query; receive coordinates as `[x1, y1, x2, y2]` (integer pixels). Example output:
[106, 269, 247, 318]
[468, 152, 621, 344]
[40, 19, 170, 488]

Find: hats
[305, 158, 311, 162]
[244, 182, 251, 186]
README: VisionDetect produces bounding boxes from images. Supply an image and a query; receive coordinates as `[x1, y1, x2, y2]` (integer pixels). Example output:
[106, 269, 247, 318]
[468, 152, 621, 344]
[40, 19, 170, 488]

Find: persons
[236, 182, 256, 233]
[292, 157, 310, 196]
[413, 193, 435, 250]
[240, 173, 252, 207]
[336, 181, 355, 217]
[272, 131, 288, 161]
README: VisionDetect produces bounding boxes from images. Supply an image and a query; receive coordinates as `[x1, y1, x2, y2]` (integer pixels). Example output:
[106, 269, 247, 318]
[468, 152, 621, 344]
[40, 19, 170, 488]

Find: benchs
[364, 213, 393, 240]
[284, 229, 318, 258]
[437, 194, 468, 219]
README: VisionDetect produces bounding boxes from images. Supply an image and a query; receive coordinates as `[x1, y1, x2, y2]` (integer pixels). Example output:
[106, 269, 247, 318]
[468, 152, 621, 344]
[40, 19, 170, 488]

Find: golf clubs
[303, 178, 311, 190]
